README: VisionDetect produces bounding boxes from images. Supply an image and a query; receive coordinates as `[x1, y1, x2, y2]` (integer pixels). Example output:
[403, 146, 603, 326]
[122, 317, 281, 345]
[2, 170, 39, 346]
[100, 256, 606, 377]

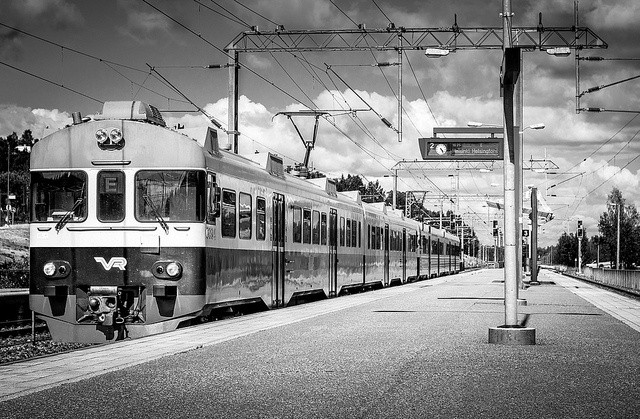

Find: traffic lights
[578, 229, 583, 236]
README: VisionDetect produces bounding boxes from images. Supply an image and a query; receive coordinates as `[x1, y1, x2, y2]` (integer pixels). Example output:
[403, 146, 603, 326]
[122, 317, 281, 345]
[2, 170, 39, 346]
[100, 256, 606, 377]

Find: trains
[28, 99, 463, 345]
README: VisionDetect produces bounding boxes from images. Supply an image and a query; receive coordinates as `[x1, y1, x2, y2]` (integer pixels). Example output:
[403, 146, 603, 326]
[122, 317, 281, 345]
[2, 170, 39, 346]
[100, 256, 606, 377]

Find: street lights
[467, 117, 545, 306]
[422, 44, 571, 346]
